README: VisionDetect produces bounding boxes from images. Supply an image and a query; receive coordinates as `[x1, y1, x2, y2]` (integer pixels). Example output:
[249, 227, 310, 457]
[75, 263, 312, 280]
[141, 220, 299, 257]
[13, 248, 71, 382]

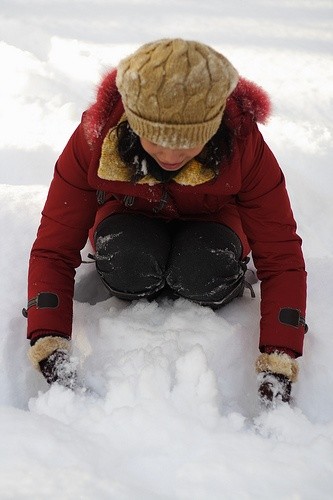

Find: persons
[20, 37, 311, 414]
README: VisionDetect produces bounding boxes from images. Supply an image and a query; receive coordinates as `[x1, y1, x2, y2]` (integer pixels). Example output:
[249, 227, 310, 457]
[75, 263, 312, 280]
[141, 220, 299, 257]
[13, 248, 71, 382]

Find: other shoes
[239, 266, 258, 287]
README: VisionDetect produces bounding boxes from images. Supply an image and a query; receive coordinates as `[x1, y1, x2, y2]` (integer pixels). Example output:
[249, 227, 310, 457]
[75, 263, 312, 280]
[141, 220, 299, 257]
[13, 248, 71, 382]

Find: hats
[112, 37, 239, 148]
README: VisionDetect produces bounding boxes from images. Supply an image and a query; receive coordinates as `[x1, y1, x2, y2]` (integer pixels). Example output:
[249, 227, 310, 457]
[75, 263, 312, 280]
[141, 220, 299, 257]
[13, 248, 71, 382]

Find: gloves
[257, 367, 297, 413]
[37, 353, 82, 392]
